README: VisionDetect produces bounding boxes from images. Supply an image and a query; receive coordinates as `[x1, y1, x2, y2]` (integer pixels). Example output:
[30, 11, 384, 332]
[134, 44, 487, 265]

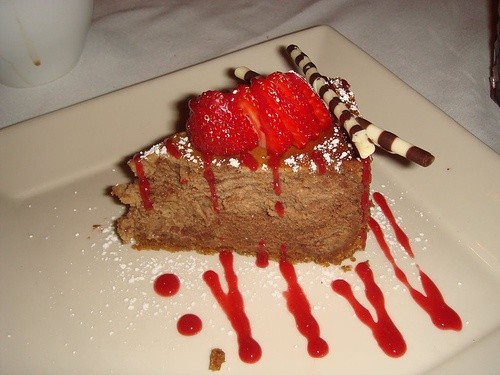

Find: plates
[1, 23, 500, 375]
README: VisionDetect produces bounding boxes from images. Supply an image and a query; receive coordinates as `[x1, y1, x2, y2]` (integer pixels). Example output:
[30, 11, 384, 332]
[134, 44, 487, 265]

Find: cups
[0, 0, 94, 89]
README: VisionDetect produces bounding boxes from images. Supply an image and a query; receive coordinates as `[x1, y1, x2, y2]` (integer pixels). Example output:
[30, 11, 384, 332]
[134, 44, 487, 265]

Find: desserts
[109, 45, 436, 267]
